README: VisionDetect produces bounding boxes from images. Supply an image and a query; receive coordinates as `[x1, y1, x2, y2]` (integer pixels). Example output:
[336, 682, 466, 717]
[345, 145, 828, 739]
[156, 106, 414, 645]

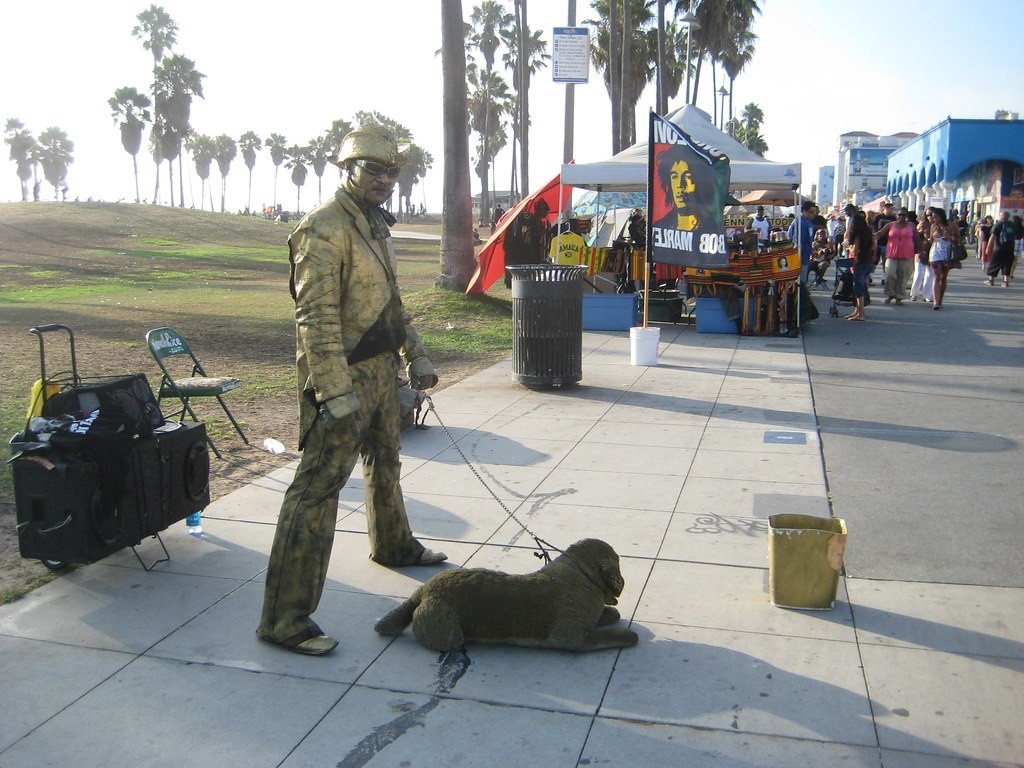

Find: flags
[648, 111, 731, 268]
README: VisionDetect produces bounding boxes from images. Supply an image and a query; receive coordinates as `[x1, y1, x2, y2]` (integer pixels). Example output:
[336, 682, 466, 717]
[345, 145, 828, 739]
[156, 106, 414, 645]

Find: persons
[254, 123, 448, 655]
[470, 204, 505, 246]
[743, 201, 1024, 321]
[628, 209, 646, 292]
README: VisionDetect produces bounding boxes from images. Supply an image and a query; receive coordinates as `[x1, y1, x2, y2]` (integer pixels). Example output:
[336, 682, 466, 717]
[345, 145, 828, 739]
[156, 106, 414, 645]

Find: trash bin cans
[767, 511, 848, 614]
[504, 262, 591, 392]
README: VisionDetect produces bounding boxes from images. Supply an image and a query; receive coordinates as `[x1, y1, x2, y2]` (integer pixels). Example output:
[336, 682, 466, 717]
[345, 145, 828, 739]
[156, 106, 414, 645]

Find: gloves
[408, 355, 438, 390]
[320, 392, 362, 438]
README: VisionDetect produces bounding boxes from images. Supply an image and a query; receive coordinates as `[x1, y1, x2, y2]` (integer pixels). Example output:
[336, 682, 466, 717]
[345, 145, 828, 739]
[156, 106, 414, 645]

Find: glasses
[807, 210, 817, 213]
[926, 213, 932, 217]
[756, 209, 763, 212]
[354, 159, 400, 178]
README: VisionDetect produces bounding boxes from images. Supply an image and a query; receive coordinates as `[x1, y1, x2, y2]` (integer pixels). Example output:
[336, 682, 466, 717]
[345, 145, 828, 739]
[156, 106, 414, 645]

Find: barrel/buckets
[629, 327, 661, 366]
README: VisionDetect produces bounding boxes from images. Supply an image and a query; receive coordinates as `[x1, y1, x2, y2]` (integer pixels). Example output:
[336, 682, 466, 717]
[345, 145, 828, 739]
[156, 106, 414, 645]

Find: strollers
[830, 234, 871, 319]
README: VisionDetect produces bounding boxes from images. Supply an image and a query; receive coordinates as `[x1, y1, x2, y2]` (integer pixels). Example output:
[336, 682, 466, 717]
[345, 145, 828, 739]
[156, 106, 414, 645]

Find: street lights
[716, 85, 731, 131]
[680, 12, 703, 105]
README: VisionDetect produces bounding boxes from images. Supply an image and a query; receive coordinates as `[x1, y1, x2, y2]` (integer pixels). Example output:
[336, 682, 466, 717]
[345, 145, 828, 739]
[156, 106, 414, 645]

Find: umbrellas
[465, 158, 606, 296]
[568, 190, 647, 240]
[736, 189, 813, 216]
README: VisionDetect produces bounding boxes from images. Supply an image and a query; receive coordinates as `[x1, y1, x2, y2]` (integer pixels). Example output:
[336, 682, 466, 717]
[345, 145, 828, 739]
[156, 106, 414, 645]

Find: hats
[756, 206, 764, 211]
[328, 125, 402, 166]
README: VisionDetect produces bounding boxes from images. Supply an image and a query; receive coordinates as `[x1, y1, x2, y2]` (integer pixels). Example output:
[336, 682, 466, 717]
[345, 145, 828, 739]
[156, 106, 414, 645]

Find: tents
[554, 104, 802, 327]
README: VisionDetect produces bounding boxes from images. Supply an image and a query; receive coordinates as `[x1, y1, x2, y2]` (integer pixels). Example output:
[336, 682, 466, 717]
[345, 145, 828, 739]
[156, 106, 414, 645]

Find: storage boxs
[582, 293, 638, 330]
[696, 297, 760, 334]
[639, 297, 683, 322]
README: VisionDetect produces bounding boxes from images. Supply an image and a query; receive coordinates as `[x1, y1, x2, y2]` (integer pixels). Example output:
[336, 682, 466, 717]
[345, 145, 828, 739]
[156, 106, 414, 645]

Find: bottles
[264, 438, 285, 454]
[186, 511, 201, 533]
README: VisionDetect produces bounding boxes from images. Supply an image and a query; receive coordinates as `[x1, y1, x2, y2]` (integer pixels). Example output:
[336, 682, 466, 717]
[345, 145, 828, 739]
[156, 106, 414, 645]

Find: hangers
[560, 222, 574, 235]
[523, 204, 530, 213]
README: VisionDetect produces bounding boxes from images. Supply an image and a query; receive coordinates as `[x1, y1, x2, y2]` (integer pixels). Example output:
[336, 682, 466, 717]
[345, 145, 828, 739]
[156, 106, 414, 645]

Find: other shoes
[418, 548, 448, 564]
[844, 313, 865, 321]
[1000, 283, 1008, 288]
[911, 296, 916, 301]
[926, 298, 934, 302]
[984, 280, 993, 286]
[257, 623, 339, 656]
[932, 305, 943, 311]
[885, 296, 903, 305]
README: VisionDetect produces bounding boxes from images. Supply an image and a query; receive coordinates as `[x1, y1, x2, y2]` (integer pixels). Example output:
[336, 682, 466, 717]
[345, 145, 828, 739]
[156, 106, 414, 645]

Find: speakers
[9, 371, 211, 566]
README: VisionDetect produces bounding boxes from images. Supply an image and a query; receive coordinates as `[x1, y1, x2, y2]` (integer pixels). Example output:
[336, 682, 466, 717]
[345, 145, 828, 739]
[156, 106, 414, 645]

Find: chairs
[146, 327, 249, 458]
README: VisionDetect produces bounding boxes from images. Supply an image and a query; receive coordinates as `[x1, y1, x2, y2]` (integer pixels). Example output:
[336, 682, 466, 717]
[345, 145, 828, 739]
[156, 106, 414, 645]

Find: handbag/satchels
[951, 240, 967, 260]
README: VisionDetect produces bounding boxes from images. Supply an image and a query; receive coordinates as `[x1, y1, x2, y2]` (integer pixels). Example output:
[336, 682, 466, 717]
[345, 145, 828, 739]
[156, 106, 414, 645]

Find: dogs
[373, 537, 640, 652]
